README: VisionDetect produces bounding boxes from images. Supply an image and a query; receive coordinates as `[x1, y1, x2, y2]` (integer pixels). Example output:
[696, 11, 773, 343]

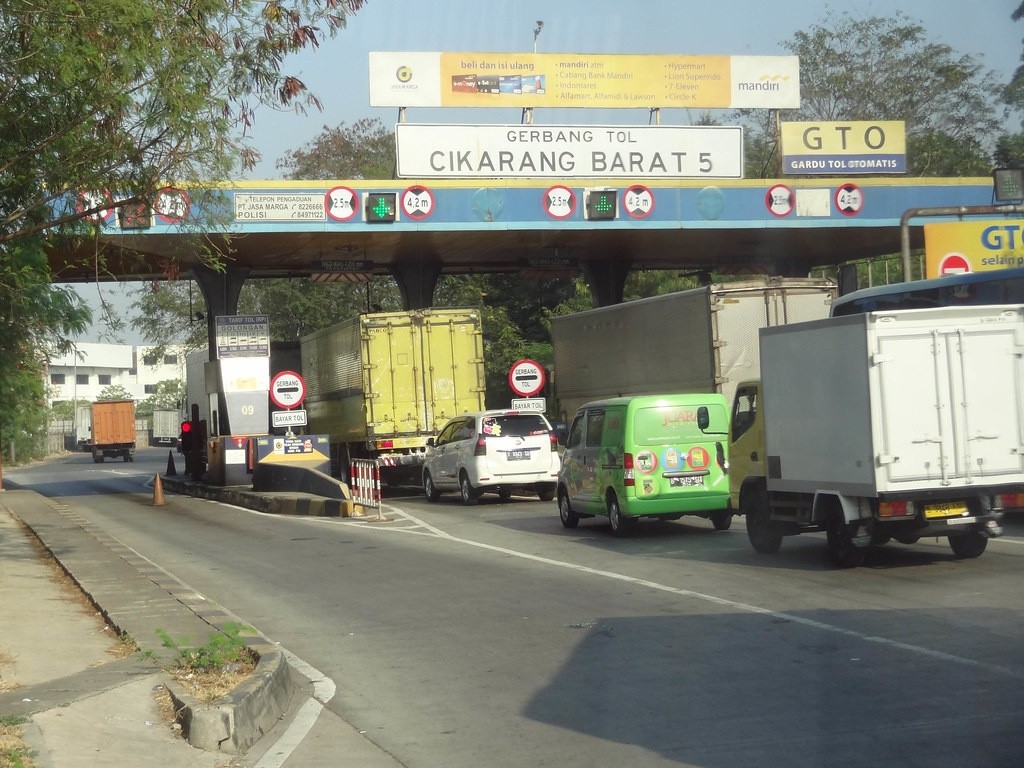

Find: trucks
[176, 342, 301, 473]
[151, 407, 188, 447]
[297, 306, 485, 488]
[89, 400, 137, 462]
[698, 302, 1024, 563]
[537, 276, 839, 457]
[77, 407, 93, 453]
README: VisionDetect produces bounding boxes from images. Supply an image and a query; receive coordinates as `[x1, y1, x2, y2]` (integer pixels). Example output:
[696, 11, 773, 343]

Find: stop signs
[944, 256, 972, 278]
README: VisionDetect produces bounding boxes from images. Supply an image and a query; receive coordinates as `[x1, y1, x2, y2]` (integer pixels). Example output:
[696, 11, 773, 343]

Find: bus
[827, 267, 1024, 316]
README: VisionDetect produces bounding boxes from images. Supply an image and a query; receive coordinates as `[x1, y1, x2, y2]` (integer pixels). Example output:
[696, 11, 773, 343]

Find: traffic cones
[150, 473, 171, 506]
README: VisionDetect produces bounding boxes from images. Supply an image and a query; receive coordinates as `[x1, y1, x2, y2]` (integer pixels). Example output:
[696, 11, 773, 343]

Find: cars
[420, 411, 561, 504]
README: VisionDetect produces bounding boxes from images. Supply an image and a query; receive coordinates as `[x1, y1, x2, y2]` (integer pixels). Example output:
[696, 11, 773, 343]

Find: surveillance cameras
[373, 305, 382, 312]
[195, 311, 205, 320]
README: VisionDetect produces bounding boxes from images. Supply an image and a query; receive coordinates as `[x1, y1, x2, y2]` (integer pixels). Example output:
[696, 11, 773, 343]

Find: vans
[554, 393, 733, 535]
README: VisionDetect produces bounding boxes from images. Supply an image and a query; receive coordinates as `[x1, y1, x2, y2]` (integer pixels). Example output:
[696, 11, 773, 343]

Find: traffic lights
[590, 194, 617, 221]
[181, 422, 191, 454]
[993, 169, 1024, 200]
[120, 199, 152, 231]
[367, 192, 397, 222]
